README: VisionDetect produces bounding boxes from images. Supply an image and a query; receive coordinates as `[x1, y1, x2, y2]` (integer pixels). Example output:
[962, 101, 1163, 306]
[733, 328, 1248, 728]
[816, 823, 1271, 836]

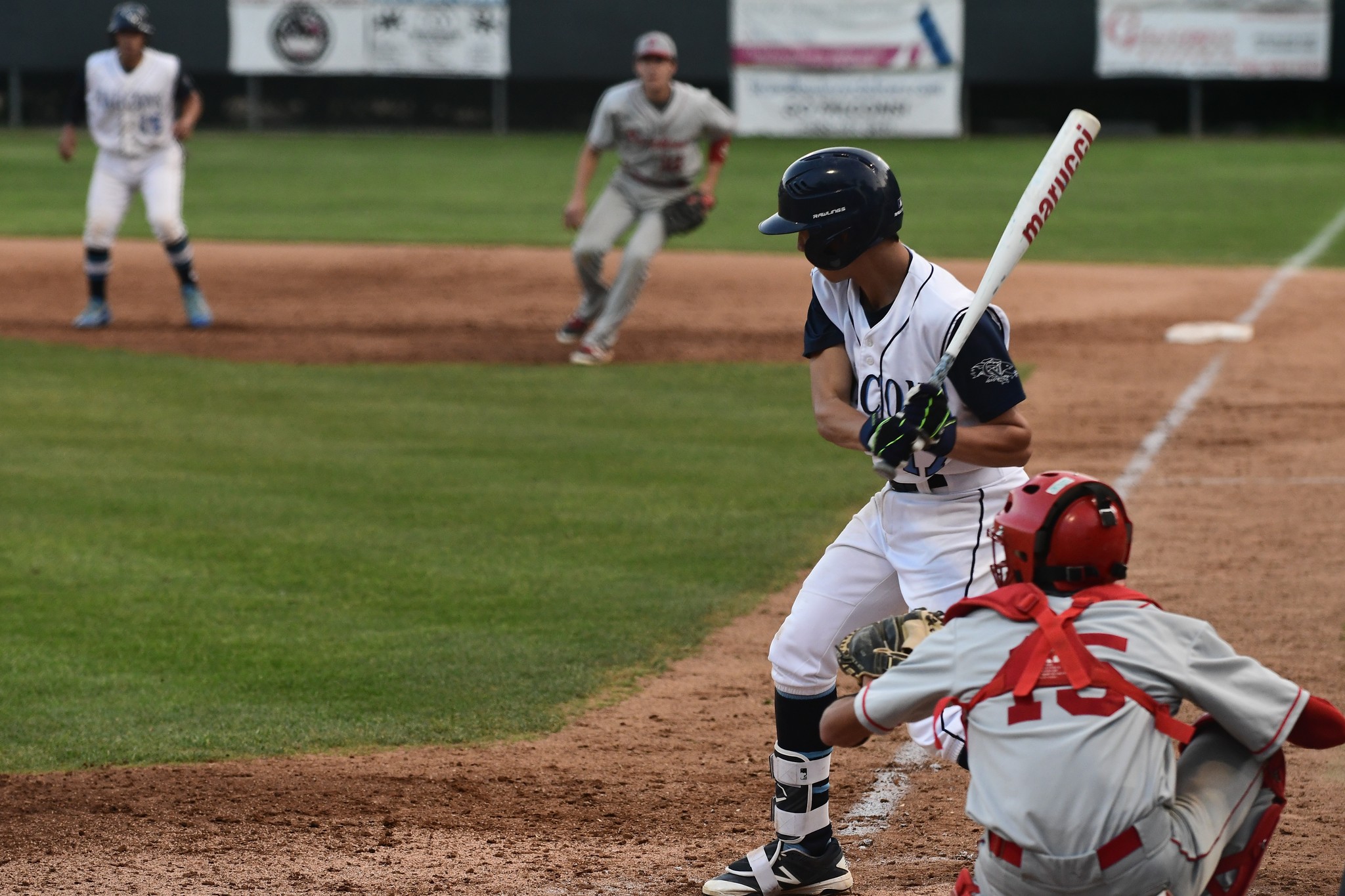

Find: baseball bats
[870, 107, 1102, 482]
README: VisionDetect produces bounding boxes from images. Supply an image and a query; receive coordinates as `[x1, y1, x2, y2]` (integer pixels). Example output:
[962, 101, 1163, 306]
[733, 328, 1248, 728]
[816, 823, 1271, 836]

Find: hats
[637, 32, 672, 59]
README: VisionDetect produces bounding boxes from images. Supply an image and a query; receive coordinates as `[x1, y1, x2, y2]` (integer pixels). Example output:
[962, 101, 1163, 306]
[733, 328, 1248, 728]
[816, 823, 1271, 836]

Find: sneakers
[702, 837, 853, 896]
[73, 299, 110, 329]
[555, 315, 588, 342]
[183, 290, 214, 327]
[570, 347, 600, 366]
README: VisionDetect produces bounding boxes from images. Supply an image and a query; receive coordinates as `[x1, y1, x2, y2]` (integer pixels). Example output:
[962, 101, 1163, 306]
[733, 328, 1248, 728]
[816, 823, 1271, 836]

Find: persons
[820, 471, 1345, 896]
[58, 11, 214, 330]
[554, 31, 734, 365]
[702, 148, 1032, 896]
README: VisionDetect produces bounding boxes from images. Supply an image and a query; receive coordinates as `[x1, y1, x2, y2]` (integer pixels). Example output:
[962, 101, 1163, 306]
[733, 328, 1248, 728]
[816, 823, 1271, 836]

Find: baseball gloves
[833, 607, 946, 686]
[661, 188, 711, 232]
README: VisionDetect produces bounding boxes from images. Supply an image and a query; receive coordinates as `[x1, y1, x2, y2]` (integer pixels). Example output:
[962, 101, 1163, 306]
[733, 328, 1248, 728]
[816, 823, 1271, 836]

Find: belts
[888, 473, 948, 492]
[990, 826, 1142, 869]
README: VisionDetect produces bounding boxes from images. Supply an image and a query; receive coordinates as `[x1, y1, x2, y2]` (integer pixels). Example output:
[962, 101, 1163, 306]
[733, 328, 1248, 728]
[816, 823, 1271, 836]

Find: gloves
[859, 412, 924, 469]
[896, 382, 958, 455]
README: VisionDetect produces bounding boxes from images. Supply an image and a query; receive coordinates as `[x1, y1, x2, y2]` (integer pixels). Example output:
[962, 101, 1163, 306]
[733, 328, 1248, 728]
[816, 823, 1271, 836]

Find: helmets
[987, 470, 1130, 594]
[109, 8, 150, 36]
[758, 147, 905, 270]
